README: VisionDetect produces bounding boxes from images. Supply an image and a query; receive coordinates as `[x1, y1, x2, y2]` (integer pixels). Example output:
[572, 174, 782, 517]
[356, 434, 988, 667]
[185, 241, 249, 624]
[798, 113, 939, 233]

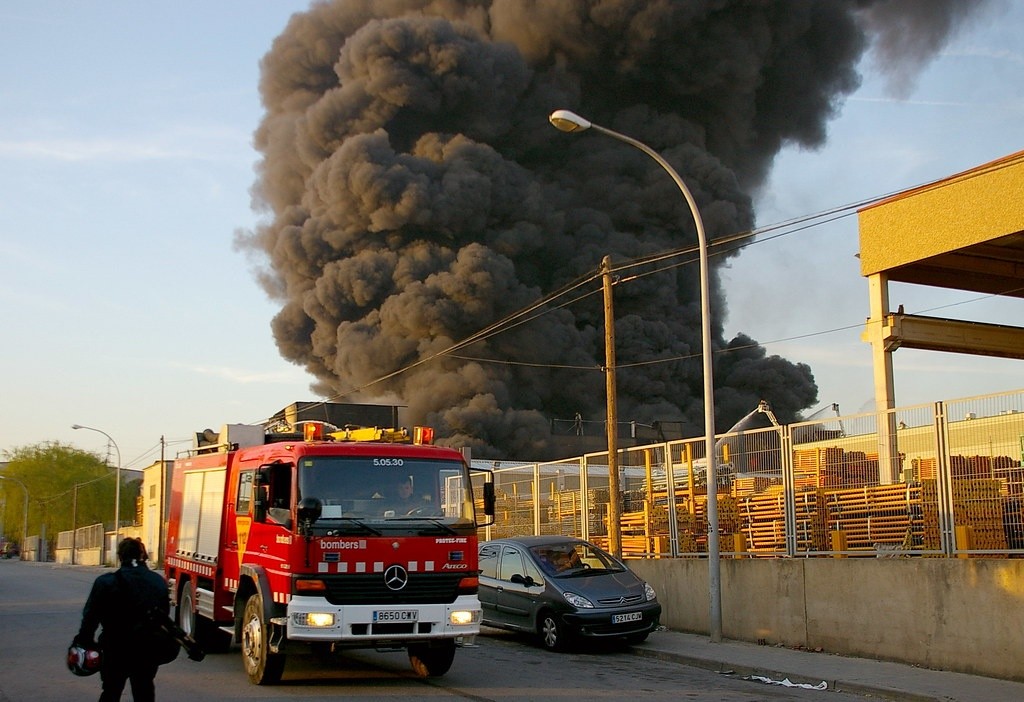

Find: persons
[73, 537, 170, 702]
[398, 476, 431, 508]
[556, 551, 573, 571]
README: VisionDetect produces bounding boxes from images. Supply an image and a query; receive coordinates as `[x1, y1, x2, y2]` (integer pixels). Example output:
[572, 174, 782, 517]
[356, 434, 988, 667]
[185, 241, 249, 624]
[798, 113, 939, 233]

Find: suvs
[0, 542, 16, 559]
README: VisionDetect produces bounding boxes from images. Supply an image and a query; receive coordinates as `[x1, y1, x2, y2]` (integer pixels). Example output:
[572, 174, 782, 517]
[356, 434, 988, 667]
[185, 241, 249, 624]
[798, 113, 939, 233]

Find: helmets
[66, 634, 104, 676]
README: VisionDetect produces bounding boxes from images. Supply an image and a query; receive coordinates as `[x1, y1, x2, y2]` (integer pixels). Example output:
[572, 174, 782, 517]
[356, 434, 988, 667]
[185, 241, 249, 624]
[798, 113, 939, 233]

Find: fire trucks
[160, 414, 497, 683]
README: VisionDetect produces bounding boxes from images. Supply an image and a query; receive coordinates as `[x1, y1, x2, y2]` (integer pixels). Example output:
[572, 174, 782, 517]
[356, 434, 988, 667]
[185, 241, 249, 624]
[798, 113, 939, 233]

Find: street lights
[72, 423, 123, 568]
[547, 108, 727, 645]
[0, 475, 33, 550]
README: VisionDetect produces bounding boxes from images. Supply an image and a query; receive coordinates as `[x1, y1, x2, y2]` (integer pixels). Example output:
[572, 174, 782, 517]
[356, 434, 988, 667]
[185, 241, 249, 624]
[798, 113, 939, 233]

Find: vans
[479, 534, 663, 653]
